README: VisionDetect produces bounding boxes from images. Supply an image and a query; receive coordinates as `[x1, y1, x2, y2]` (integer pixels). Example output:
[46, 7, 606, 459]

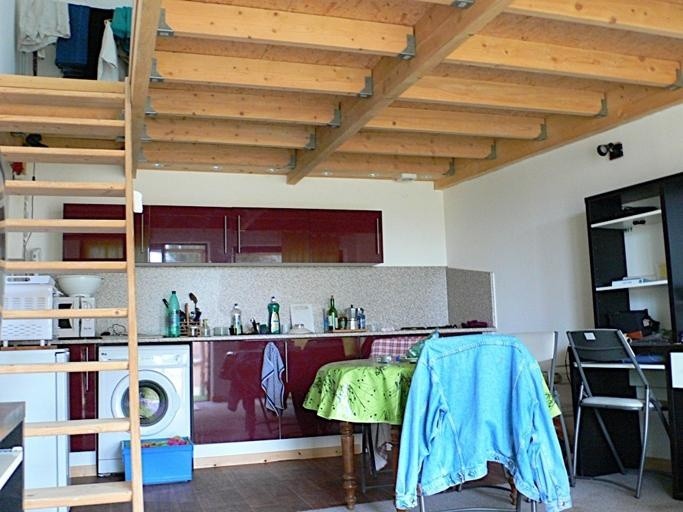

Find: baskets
[179, 320, 201, 335]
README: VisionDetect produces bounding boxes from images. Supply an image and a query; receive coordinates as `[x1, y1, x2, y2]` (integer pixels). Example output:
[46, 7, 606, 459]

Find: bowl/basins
[55, 275, 103, 297]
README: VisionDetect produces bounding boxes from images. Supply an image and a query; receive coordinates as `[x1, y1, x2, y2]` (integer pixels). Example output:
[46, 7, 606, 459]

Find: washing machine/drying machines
[98, 345, 192, 474]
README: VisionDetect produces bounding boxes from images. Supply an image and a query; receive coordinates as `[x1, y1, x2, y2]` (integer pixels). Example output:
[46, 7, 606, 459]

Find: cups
[188, 326, 198, 338]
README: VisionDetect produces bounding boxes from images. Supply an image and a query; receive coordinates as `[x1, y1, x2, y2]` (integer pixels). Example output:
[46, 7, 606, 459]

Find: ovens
[2, 283, 55, 348]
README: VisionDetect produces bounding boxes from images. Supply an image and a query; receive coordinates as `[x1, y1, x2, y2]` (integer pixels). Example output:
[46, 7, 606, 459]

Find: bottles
[328, 295, 366, 330]
[233, 301, 242, 335]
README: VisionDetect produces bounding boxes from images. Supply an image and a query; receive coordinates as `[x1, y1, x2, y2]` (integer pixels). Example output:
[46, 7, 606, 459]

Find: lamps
[597, 143, 623, 160]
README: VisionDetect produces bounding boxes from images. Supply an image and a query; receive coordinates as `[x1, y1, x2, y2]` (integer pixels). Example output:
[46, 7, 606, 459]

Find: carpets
[305, 463, 683, 512]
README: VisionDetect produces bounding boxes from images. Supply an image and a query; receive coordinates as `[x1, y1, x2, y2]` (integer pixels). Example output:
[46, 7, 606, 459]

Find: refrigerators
[0, 349, 70, 512]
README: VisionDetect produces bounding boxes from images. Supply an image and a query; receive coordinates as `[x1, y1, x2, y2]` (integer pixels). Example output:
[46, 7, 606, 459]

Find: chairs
[567, 329, 671, 499]
[371, 330, 577, 511]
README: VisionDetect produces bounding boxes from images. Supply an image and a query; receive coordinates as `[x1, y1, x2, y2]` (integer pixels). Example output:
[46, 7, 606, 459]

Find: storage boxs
[120, 437, 193, 488]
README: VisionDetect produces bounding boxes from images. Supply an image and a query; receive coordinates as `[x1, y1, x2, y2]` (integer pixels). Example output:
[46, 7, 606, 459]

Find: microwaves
[54, 297, 95, 338]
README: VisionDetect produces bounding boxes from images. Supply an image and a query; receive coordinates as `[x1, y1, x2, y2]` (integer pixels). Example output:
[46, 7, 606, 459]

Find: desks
[317, 357, 420, 509]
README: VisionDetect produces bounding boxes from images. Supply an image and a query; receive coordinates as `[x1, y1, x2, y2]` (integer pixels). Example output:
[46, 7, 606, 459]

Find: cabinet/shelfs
[586, 174, 683, 343]
[61, 206, 383, 267]
[190, 336, 370, 443]
[56, 345, 96, 452]
